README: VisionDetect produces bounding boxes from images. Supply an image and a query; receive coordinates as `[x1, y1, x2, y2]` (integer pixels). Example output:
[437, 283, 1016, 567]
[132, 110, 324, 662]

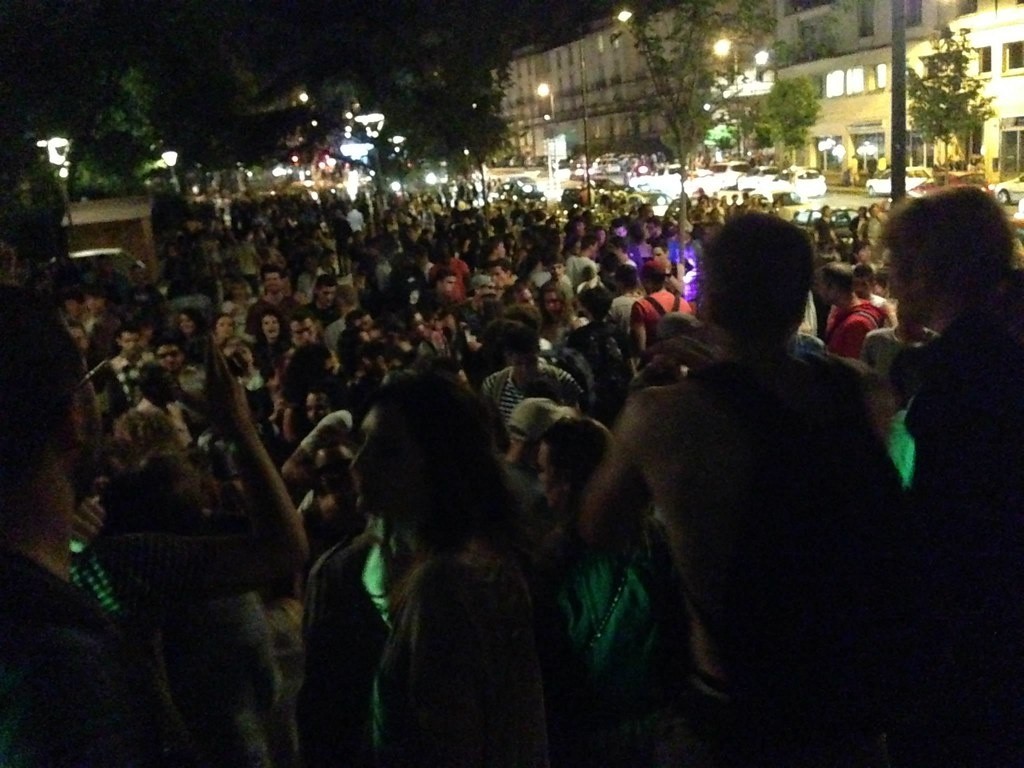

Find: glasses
[290, 325, 315, 338]
[69, 356, 109, 392]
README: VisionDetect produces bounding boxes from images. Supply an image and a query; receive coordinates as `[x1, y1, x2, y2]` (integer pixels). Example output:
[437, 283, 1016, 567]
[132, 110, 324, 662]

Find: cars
[907, 171, 992, 196]
[496, 152, 827, 199]
[865, 169, 930, 196]
[993, 174, 1023, 204]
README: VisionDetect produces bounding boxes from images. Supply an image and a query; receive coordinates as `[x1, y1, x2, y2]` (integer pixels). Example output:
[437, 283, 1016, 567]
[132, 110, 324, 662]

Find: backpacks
[581, 324, 628, 402]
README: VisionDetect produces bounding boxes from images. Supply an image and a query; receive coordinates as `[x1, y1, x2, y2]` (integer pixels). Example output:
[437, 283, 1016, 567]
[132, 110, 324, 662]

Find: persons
[2, 165, 1024, 768]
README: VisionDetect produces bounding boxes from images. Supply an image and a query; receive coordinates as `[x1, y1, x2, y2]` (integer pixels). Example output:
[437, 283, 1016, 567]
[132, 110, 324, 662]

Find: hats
[471, 274, 495, 287]
[509, 397, 578, 445]
[658, 311, 704, 337]
